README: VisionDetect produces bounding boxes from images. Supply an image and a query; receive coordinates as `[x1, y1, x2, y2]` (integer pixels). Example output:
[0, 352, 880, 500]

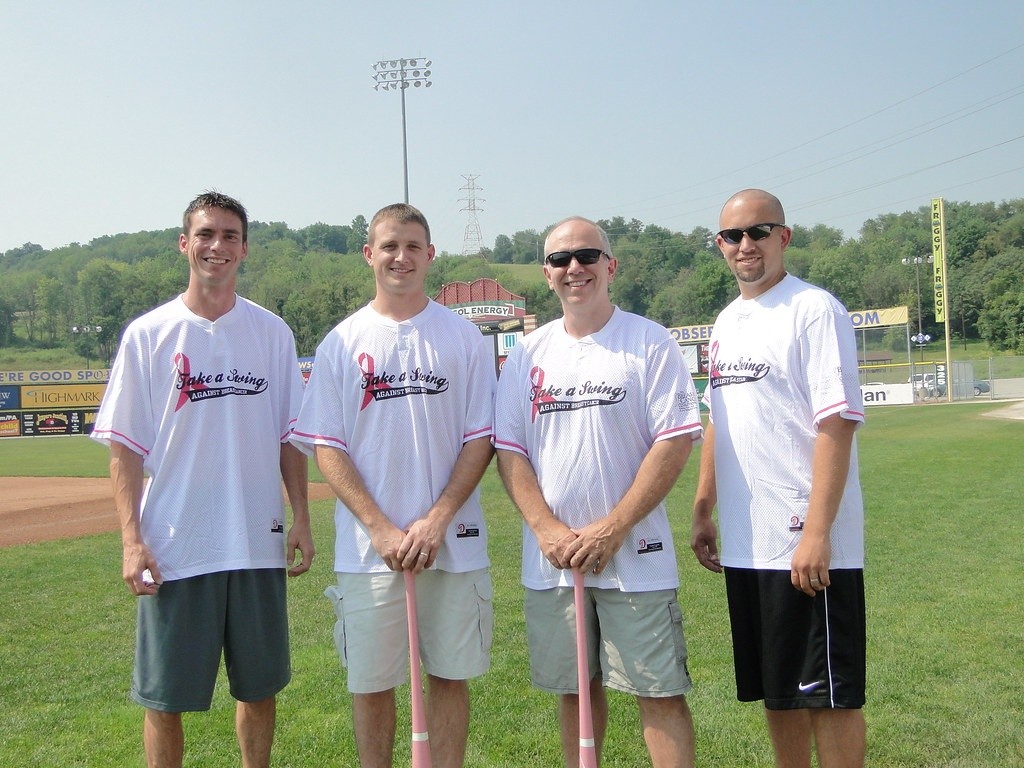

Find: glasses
[716, 223, 784, 245]
[545, 248, 611, 268]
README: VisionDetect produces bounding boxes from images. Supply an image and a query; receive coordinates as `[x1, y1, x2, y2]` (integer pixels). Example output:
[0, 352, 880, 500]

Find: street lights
[960, 296, 970, 351]
[370, 58, 434, 206]
[901, 253, 935, 399]
[72, 324, 102, 371]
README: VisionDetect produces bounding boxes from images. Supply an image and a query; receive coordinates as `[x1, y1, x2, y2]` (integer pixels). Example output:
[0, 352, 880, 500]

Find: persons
[692, 188, 867, 768]
[89, 193, 314, 768]
[288, 204, 496, 768]
[490, 217, 704, 768]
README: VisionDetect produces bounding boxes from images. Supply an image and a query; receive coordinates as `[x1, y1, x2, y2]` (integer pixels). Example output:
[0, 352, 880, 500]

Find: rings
[420, 552, 429, 557]
[810, 578, 819, 582]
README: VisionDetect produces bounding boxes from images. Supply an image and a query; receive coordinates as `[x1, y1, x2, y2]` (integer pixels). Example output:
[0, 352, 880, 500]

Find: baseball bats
[401, 529, 433, 768]
[571, 567, 598, 768]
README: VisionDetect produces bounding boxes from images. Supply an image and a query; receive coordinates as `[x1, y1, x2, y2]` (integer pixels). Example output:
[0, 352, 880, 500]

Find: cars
[907, 373, 990, 398]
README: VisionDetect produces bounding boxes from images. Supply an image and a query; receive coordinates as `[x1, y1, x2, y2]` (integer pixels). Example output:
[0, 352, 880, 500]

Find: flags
[930, 199, 945, 322]
